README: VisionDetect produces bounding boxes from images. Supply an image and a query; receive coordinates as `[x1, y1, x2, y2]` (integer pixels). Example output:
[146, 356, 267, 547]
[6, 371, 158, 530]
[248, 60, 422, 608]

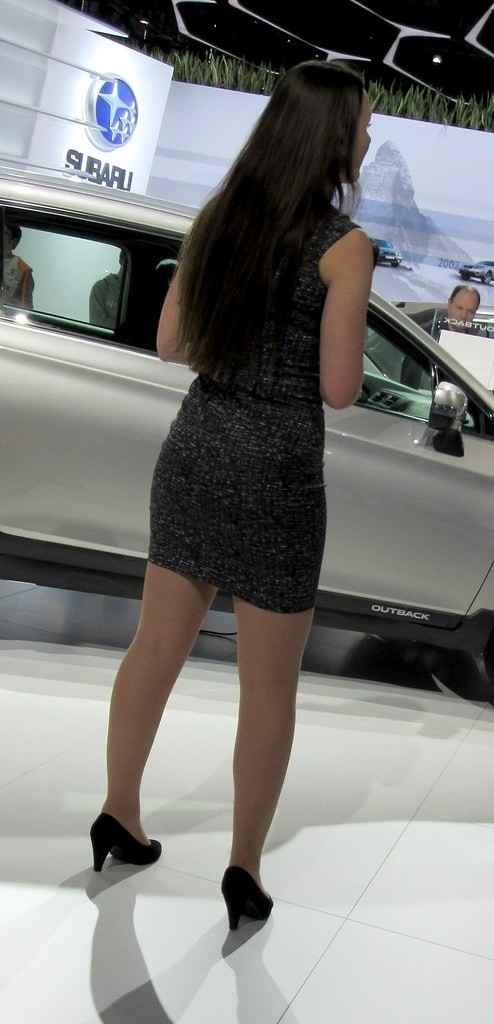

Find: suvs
[0, 172, 494, 704]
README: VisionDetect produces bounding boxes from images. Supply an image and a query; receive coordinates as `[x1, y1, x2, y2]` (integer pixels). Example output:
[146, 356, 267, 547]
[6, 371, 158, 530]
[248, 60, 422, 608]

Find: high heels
[221, 865, 273, 931]
[89, 813, 162, 872]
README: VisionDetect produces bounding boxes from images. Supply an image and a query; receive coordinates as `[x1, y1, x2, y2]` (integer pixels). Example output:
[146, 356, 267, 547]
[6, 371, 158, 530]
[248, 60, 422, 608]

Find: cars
[461, 261, 494, 284]
[369, 236, 402, 267]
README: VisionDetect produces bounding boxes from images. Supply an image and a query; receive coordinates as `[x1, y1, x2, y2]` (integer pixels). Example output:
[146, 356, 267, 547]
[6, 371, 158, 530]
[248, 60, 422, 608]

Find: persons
[401, 285, 488, 390]
[3, 222, 35, 312]
[89, 249, 125, 328]
[90, 62, 372, 928]
